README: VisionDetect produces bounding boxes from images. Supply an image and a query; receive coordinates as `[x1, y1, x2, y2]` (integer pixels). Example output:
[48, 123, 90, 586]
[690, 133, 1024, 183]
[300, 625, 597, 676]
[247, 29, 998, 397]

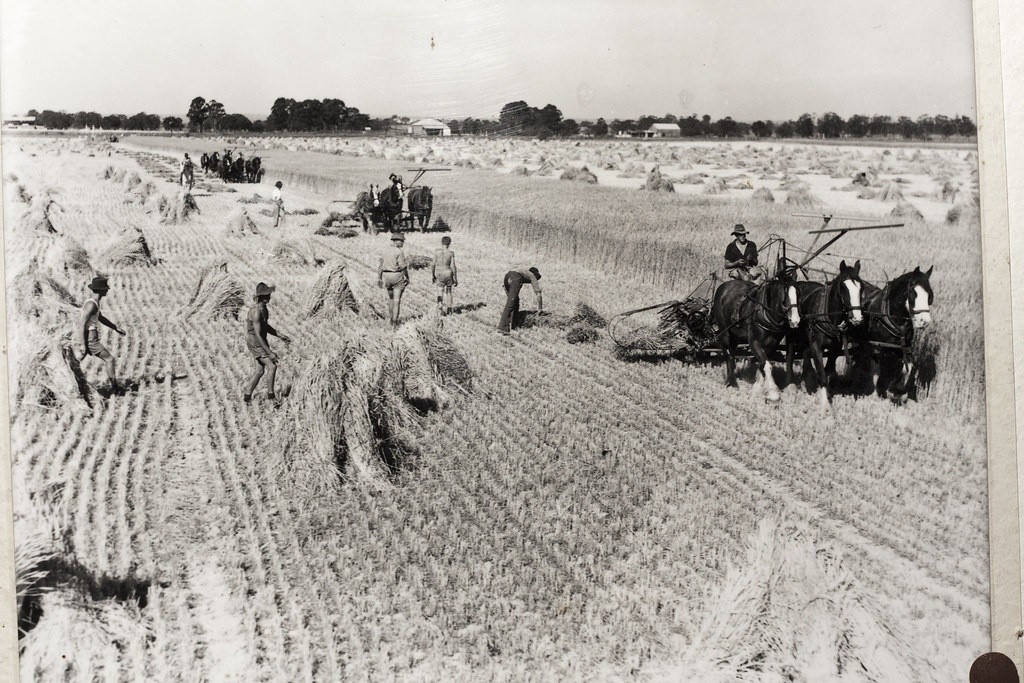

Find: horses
[782, 259, 867, 409]
[351, 178, 433, 236]
[712, 256, 803, 409]
[200, 152, 261, 184]
[847, 264, 935, 407]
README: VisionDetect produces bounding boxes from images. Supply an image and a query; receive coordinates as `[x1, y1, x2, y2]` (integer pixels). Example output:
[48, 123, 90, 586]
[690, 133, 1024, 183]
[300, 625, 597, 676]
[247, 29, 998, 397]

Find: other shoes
[498, 326, 511, 336]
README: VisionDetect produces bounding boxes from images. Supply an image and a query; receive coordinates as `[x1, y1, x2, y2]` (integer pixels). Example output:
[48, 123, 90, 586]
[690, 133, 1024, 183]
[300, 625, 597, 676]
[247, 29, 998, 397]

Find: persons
[431, 236, 459, 316]
[387, 173, 398, 191]
[378, 233, 410, 332]
[73, 277, 126, 396]
[724, 224, 766, 288]
[244, 282, 291, 402]
[180, 152, 193, 168]
[270, 180, 286, 227]
[497, 266, 542, 335]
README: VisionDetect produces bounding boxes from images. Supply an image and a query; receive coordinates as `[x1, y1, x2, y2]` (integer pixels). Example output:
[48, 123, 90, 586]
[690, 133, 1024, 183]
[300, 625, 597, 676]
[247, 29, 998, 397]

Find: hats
[530, 267, 543, 284]
[253, 283, 277, 301]
[390, 231, 407, 243]
[87, 275, 111, 293]
[730, 225, 750, 239]
